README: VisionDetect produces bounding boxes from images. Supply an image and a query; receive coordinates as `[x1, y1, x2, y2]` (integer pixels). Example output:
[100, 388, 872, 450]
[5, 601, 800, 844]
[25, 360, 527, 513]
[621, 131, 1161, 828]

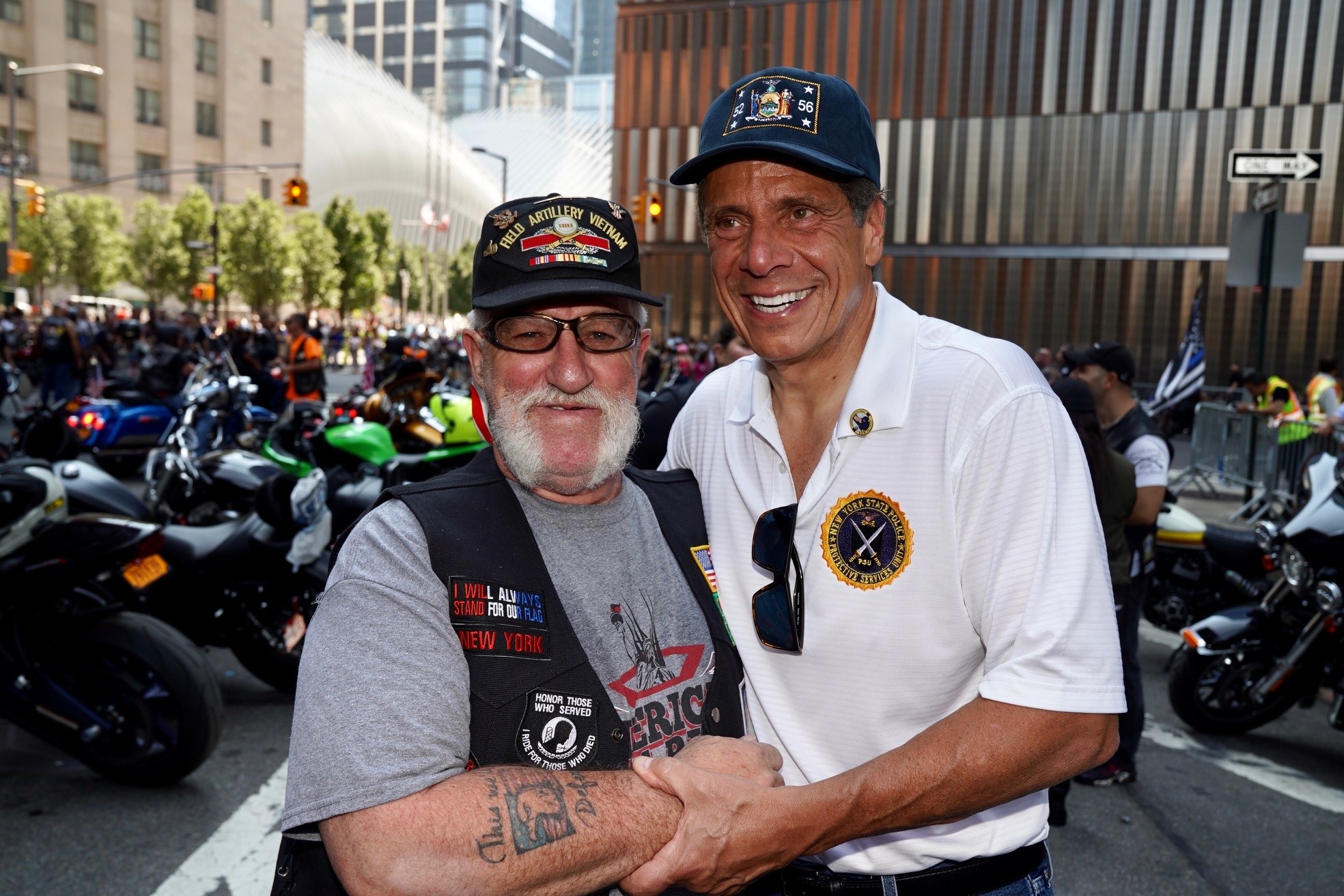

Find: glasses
[477, 312, 640, 354]
[752, 504, 805, 653]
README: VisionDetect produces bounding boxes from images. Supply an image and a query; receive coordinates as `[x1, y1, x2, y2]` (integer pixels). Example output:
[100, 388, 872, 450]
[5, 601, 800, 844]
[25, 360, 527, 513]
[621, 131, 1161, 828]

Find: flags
[1146, 289, 1209, 417]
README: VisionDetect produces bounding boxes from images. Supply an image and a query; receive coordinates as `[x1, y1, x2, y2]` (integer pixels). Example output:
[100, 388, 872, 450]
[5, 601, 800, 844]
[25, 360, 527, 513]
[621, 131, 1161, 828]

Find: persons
[616, 66, 1130, 896]
[270, 192, 787, 896]
[0, 301, 1344, 789]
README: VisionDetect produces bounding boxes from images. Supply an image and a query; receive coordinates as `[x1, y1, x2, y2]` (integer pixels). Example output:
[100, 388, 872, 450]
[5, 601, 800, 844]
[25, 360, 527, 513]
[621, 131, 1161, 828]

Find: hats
[670, 67, 881, 185]
[473, 193, 663, 308]
[1064, 342, 1134, 385]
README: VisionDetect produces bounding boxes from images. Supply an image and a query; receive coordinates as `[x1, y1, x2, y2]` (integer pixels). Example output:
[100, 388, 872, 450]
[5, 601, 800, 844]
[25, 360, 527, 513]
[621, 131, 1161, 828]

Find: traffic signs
[1228, 148, 1324, 184]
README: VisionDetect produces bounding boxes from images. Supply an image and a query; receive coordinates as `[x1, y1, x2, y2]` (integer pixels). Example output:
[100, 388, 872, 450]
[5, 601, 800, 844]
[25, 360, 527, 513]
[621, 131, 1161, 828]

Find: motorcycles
[1132, 448, 1344, 736]
[0, 349, 493, 789]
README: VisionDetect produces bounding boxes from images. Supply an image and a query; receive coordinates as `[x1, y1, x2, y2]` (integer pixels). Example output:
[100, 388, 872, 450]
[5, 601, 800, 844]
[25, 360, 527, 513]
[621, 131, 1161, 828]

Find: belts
[758, 842, 1046, 896]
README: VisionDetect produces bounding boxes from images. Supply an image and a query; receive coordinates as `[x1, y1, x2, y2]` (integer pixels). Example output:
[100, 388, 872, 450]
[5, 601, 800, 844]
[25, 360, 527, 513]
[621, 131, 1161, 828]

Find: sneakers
[1074, 763, 1136, 786]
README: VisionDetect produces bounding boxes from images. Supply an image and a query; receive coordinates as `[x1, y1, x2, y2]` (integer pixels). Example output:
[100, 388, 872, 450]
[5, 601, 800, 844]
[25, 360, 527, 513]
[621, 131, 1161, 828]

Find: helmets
[0, 460, 66, 558]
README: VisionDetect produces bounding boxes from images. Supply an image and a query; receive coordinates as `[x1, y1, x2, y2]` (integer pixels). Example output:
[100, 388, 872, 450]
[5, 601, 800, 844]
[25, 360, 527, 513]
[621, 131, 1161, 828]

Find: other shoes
[1046, 801, 1067, 826]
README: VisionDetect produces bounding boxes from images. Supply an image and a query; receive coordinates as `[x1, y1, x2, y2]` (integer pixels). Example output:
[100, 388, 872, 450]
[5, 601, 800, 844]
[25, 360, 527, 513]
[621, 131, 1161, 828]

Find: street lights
[6, 62, 104, 288]
[471, 147, 508, 204]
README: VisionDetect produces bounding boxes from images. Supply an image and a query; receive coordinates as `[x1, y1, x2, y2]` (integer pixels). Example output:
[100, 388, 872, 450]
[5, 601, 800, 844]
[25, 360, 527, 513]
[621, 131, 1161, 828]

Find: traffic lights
[7, 248, 33, 275]
[192, 284, 216, 304]
[630, 193, 642, 226]
[283, 177, 307, 207]
[26, 182, 47, 221]
[651, 194, 661, 226]
